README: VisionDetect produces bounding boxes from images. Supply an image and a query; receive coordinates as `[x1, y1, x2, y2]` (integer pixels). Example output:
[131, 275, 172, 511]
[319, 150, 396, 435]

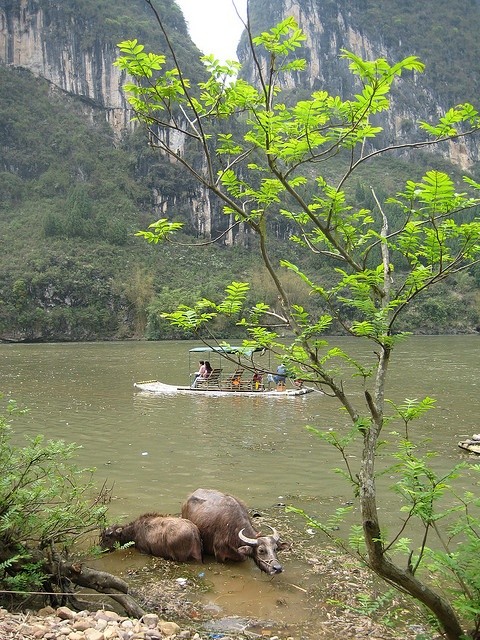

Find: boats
[459, 432, 478, 452]
[133, 346, 315, 398]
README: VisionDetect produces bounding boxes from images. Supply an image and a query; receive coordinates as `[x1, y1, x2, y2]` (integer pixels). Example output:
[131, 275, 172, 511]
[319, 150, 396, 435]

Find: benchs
[242, 370, 265, 391]
[195, 368, 223, 389]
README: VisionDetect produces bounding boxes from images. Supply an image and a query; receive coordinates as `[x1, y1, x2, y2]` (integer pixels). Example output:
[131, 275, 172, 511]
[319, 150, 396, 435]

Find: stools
[275, 380, 287, 393]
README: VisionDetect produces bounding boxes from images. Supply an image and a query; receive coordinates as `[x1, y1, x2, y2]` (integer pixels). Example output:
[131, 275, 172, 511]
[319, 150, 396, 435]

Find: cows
[183, 487, 285, 577]
[99, 513, 204, 565]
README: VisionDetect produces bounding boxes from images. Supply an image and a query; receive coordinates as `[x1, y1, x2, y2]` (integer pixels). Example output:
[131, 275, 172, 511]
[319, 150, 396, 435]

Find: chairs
[227, 369, 244, 389]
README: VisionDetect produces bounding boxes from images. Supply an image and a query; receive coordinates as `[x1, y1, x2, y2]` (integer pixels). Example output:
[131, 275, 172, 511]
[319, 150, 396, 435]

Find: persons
[193, 361, 205, 381]
[191, 361, 213, 388]
[273, 361, 286, 385]
